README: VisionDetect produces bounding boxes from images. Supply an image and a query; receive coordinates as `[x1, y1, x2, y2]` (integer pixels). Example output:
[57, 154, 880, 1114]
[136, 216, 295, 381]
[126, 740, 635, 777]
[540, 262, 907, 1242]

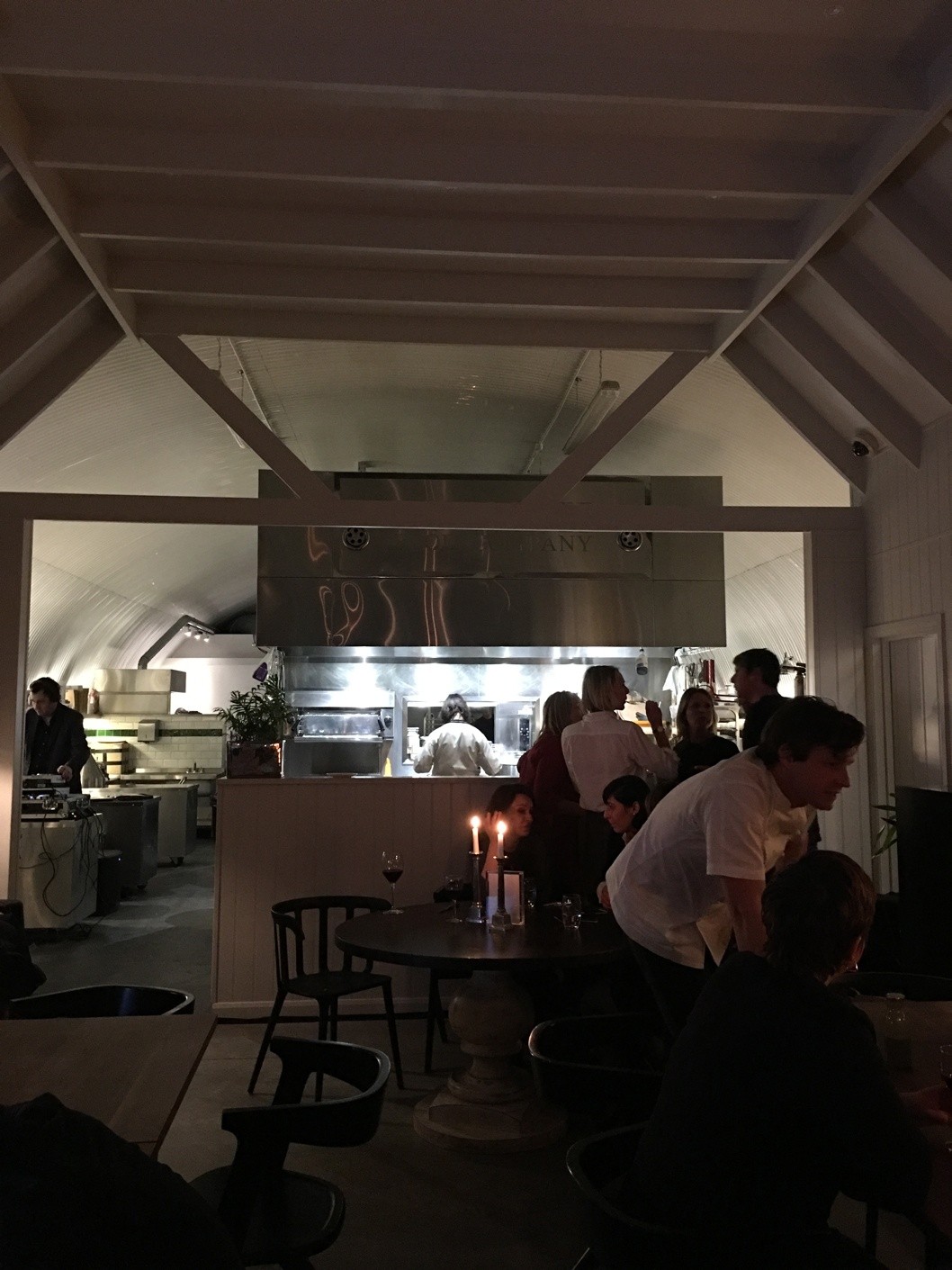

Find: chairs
[527, 1013, 685, 1270]
[247, 894, 405, 1104]
[827, 971, 952, 1001]
[425, 882, 487, 1075]
[0, 984, 195, 1015]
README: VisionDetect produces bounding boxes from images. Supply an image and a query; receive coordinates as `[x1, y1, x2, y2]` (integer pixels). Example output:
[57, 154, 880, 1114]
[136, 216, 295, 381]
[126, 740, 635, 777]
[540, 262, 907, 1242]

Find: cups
[562, 894, 581, 930]
[884, 993, 907, 1029]
[522, 878, 537, 908]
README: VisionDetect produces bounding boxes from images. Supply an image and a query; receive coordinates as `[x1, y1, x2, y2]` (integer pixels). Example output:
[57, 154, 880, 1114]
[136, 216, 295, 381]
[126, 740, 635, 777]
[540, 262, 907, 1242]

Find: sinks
[120, 773, 224, 796]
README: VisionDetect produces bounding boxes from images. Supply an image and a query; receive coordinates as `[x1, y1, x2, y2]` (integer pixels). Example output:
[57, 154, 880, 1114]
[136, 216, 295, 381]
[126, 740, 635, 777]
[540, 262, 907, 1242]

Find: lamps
[561, 349, 621, 458]
[203, 346, 262, 450]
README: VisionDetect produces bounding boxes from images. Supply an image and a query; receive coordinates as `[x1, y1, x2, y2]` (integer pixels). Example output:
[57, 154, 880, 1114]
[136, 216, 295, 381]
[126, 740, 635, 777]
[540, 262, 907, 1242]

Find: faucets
[192, 762, 198, 773]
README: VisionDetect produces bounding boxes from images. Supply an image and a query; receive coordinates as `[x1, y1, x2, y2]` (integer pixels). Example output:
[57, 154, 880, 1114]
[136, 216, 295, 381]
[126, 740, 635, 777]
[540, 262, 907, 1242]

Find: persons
[606, 695, 865, 1044]
[415, 694, 504, 776]
[467, 648, 820, 1020]
[24, 677, 91, 794]
[633, 851, 952, 1270]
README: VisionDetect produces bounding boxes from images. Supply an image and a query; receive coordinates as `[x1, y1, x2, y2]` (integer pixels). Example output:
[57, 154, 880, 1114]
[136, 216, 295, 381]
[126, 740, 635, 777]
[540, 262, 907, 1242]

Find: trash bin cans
[93, 849, 121, 917]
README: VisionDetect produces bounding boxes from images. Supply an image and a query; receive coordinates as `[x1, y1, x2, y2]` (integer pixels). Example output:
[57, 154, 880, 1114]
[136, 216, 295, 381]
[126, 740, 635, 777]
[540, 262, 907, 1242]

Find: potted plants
[214, 670, 309, 778]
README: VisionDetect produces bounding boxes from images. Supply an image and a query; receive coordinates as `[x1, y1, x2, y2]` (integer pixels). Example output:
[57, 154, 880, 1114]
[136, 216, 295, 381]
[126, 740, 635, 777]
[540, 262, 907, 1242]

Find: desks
[0, 1014, 218, 1159]
[827, 998, 952, 1270]
[332, 902, 628, 1139]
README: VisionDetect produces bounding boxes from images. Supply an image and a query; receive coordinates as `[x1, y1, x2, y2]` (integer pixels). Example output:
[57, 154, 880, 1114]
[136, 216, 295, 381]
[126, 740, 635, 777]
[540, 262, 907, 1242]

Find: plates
[326, 773, 358, 778]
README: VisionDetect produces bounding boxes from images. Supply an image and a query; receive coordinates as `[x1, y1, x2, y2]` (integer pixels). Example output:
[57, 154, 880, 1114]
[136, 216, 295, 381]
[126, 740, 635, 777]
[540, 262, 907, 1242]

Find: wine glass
[940, 1044, 952, 1154]
[446, 875, 464, 923]
[381, 850, 406, 915]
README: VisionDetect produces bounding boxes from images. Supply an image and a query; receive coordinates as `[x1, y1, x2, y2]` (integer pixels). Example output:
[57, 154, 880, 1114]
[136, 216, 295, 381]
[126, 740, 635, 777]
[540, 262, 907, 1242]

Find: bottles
[636, 649, 648, 675]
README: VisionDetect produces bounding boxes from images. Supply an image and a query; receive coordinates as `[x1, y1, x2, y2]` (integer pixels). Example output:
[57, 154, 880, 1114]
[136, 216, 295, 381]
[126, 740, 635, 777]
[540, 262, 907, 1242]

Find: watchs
[653, 726, 664, 733]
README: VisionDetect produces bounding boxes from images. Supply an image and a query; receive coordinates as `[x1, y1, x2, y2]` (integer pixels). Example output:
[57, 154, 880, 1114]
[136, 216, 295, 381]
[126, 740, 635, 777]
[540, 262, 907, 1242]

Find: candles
[493, 819, 509, 857]
[469, 815, 482, 854]
